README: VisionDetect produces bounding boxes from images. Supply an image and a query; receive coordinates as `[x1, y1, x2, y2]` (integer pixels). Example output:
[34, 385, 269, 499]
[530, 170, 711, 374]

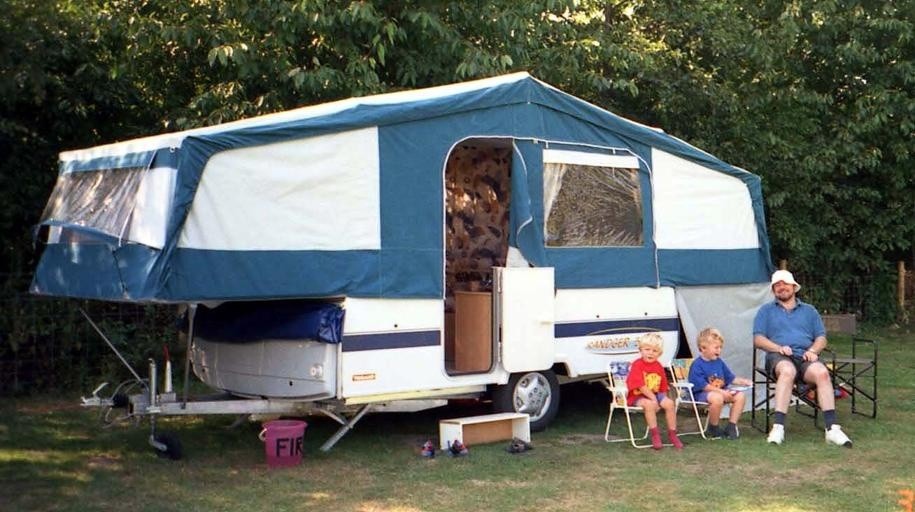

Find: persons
[686, 328, 752, 439]
[753, 270, 853, 449]
[625, 331, 684, 448]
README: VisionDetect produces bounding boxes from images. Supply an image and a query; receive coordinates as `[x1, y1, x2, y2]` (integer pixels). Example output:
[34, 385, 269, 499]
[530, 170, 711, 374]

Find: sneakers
[421, 441, 435, 458]
[767, 424, 785, 445]
[704, 423, 726, 440]
[724, 422, 739, 440]
[448, 440, 467, 456]
[825, 424, 852, 449]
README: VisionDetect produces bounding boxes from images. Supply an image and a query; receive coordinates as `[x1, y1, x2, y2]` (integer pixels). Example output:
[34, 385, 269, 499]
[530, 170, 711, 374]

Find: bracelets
[808, 349, 817, 354]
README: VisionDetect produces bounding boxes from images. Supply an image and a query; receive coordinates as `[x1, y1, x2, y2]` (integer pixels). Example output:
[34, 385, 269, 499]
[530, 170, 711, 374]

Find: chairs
[604, 358, 751, 449]
[746, 327, 836, 434]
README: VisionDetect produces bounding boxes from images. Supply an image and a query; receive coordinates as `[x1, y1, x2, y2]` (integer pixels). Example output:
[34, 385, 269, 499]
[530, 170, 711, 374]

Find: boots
[650, 427, 662, 449]
[667, 430, 683, 448]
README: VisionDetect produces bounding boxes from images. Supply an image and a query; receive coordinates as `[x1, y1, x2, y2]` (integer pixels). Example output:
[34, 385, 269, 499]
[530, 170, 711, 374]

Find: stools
[795, 336, 878, 420]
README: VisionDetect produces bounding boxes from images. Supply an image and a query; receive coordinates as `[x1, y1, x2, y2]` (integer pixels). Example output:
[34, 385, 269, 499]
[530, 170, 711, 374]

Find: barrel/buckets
[258, 420, 307, 468]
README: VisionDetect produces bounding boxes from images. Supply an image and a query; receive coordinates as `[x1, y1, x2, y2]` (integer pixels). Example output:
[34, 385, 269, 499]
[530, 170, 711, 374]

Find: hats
[768, 270, 801, 293]
